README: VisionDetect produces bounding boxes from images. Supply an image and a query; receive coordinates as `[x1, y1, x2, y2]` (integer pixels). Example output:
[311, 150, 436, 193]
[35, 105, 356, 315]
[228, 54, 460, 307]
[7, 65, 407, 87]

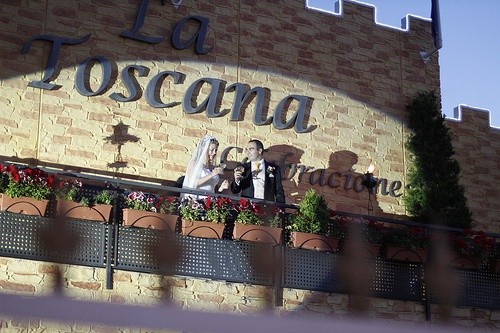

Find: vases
[232, 222, 283, 244]
[436, 250, 477, 269]
[56, 199, 113, 221]
[122, 208, 178, 231]
[344, 240, 379, 259]
[386, 247, 427, 263]
[0, 193, 49, 216]
[182, 219, 225, 239]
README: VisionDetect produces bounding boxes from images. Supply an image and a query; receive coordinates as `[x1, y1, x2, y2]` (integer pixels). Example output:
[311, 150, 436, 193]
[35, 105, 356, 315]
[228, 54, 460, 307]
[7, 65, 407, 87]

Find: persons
[182, 135, 230, 204]
[231, 140, 285, 203]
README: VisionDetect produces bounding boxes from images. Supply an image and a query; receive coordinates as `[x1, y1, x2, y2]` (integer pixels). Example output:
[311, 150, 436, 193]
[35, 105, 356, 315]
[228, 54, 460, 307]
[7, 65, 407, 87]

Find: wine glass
[236, 166, 244, 178]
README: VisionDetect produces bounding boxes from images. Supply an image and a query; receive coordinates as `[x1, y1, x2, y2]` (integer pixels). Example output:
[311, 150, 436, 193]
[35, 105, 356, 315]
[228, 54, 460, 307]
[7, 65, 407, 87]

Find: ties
[253, 162, 260, 174]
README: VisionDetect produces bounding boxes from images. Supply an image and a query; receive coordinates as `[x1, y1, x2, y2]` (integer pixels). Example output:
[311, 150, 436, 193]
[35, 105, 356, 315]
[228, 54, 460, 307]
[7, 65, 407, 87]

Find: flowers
[127, 191, 177, 214]
[345, 222, 382, 238]
[54, 175, 116, 207]
[177, 195, 232, 227]
[0, 163, 52, 201]
[386, 229, 428, 245]
[443, 231, 490, 253]
[233, 198, 285, 226]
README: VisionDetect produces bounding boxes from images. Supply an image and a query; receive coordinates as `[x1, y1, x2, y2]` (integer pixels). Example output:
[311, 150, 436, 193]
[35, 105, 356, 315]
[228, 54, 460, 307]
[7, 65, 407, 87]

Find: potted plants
[288, 189, 341, 254]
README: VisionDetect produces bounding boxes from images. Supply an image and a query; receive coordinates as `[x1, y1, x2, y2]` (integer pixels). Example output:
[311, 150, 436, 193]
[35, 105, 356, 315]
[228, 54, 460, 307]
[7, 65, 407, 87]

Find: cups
[219, 163, 227, 168]
